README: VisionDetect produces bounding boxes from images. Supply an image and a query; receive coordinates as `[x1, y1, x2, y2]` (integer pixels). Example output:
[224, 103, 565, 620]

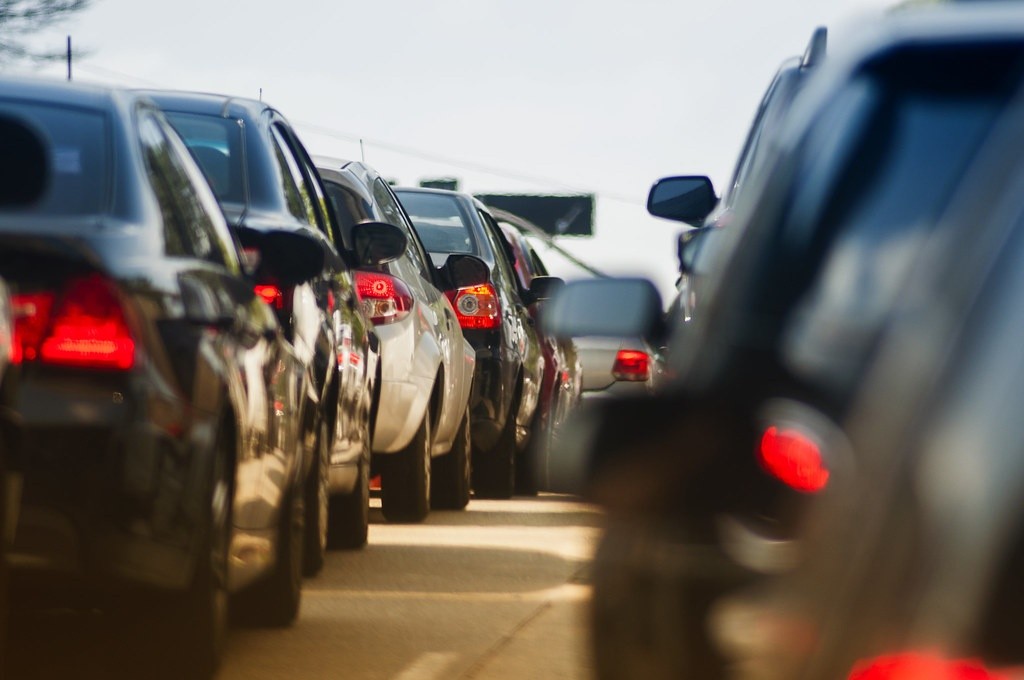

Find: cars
[0, 76, 343, 680]
[535, 0, 1023, 678]
[132, 90, 668, 576]
